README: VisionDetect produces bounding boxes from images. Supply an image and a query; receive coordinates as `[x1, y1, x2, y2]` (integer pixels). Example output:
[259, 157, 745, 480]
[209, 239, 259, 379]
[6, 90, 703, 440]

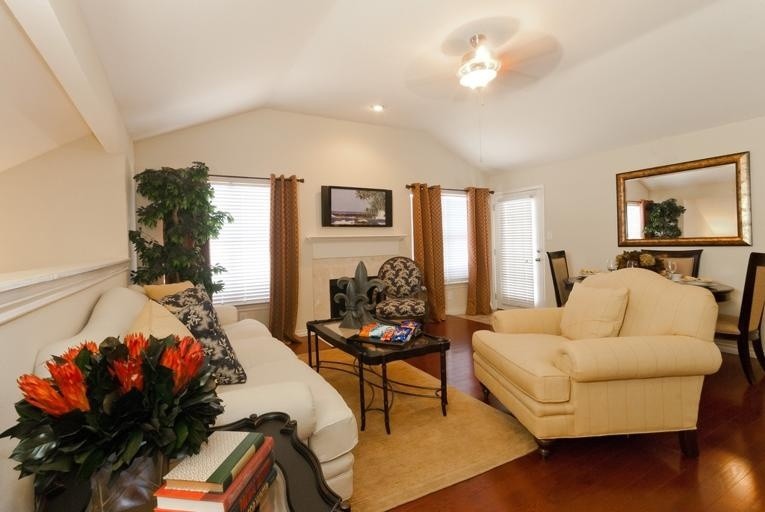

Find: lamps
[454, 30, 506, 92]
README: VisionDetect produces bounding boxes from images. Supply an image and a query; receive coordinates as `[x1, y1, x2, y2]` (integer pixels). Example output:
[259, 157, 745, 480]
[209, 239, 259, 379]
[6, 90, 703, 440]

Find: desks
[160, 408, 351, 512]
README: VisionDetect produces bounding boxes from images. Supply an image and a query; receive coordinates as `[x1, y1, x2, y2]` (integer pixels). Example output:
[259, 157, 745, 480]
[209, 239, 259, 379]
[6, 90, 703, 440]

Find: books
[151, 430, 277, 512]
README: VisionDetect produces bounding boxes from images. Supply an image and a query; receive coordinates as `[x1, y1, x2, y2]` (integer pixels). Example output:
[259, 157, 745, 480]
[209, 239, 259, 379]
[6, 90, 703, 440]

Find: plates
[683, 277, 713, 287]
[576, 272, 598, 278]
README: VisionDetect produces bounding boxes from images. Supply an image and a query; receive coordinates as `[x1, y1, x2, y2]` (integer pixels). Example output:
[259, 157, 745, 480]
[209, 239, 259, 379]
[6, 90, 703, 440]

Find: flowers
[1, 319, 227, 510]
[614, 249, 667, 275]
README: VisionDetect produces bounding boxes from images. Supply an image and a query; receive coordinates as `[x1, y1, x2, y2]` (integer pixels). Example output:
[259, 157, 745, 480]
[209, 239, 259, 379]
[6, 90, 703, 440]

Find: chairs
[711, 247, 765, 389]
[545, 247, 572, 309]
[371, 254, 431, 335]
[637, 247, 706, 279]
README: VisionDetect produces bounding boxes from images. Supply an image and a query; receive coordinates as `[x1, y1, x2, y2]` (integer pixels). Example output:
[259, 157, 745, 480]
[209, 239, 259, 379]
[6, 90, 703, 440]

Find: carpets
[285, 336, 550, 512]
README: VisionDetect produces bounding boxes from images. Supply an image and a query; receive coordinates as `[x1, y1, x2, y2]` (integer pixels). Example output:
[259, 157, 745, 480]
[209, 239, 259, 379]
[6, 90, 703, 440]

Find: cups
[606, 257, 619, 272]
[626, 260, 638, 268]
[671, 272, 682, 282]
[664, 260, 678, 274]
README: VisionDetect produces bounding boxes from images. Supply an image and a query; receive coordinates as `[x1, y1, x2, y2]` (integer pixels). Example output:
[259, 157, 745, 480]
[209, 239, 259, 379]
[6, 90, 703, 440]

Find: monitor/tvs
[321, 186, 392, 227]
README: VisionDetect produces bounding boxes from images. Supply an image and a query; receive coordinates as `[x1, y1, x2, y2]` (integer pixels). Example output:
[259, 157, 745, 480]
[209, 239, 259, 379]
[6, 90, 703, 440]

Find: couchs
[466, 261, 728, 469]
[28, 274, 366, 512]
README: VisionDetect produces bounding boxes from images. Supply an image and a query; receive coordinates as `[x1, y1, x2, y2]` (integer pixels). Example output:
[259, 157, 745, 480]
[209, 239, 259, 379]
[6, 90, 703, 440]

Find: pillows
[556, 277, 632, 345]
[140, 277, 196, 306]
[155, 281, 248, 387]
[120, 294, 199, 347]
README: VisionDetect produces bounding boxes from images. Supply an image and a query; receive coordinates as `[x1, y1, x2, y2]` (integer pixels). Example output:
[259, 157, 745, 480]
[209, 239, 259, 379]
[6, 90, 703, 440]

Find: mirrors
[609, 149, 756, 245]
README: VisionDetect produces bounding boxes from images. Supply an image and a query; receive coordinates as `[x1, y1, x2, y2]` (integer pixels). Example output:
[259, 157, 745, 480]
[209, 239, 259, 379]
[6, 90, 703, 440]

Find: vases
[78, 430, 170, 511]
